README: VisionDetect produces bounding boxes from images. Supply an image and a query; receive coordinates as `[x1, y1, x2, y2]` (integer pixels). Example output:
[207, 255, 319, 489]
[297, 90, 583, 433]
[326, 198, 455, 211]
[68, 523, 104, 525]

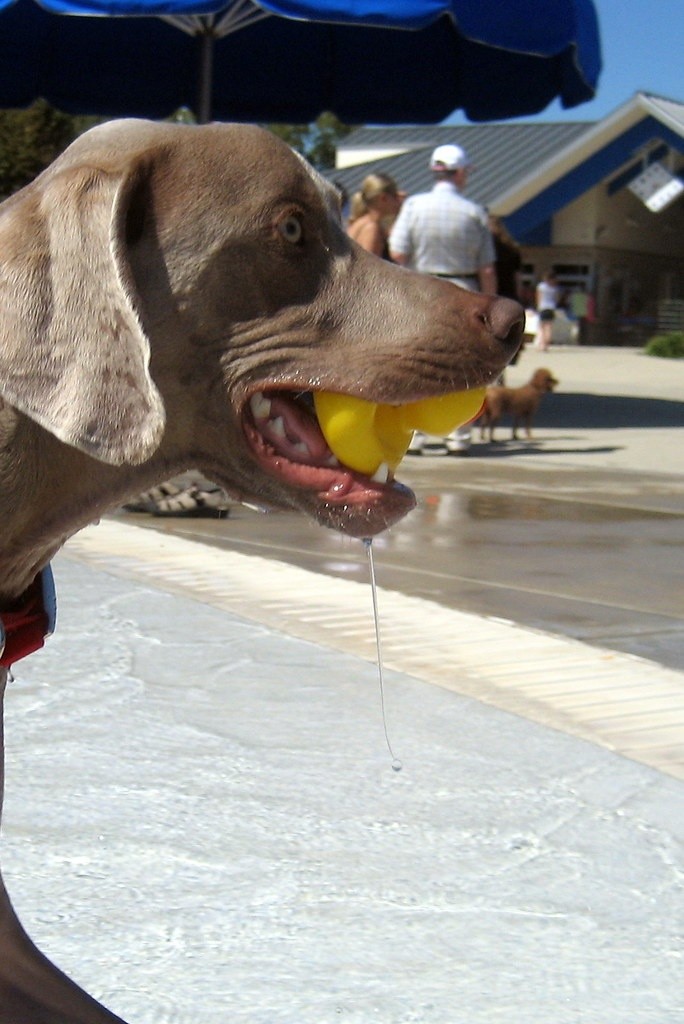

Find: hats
[428, 144, 479, 171]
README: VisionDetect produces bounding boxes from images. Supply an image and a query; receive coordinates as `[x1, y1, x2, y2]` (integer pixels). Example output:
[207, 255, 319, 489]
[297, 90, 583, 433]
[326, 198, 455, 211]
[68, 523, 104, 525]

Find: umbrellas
[1, 1, 604, 128]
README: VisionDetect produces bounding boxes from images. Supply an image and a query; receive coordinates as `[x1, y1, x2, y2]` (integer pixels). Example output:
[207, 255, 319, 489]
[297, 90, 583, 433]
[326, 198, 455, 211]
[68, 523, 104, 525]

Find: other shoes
[406, 435, 422, 455]
[445, 438, 471, 456]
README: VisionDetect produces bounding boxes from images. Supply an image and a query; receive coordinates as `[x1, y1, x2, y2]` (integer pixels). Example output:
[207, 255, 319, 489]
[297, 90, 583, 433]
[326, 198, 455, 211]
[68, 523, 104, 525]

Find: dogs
[478, 367, 559, 443]
[1, 115, 527, 1024]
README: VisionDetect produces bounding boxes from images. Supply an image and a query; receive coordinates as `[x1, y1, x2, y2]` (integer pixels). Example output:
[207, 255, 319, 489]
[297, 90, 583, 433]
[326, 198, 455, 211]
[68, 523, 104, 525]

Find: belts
[429, 274, 478, 278]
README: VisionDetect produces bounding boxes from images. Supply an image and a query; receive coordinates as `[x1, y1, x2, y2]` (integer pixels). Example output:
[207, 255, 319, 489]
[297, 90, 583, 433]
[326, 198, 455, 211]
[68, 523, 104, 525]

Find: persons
[121, 472, 234, 520]
[342, 142, 590, 459]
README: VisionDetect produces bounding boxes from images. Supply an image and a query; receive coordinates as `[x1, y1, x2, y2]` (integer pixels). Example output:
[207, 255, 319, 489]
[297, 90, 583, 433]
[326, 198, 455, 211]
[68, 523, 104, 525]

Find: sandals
[122, 481, 182, 512]
[149, 486, 230, 518]
